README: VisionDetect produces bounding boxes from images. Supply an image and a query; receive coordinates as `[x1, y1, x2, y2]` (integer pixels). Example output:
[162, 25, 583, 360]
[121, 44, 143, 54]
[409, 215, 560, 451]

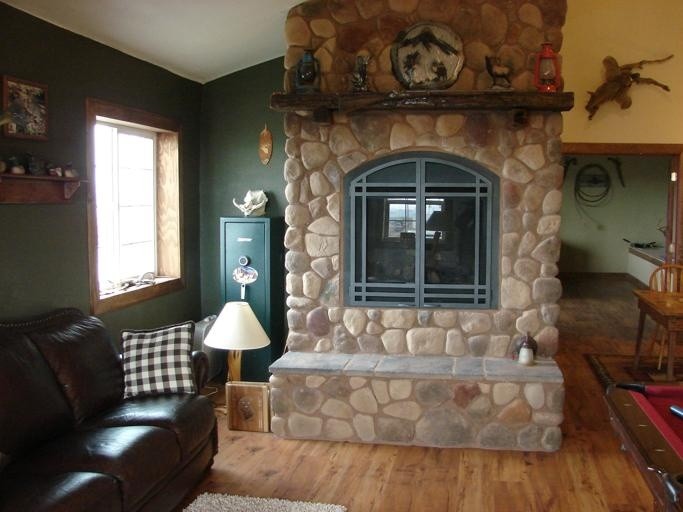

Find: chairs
[648, 264, 683, 370]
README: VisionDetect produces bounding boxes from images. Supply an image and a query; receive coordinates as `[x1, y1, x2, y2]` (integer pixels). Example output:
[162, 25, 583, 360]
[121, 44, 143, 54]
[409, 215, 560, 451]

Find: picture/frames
[2, 74, 50, 141]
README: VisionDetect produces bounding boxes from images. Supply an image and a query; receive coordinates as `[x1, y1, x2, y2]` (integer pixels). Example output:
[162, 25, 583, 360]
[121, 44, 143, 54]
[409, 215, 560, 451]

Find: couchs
[0, 308, 218, 512]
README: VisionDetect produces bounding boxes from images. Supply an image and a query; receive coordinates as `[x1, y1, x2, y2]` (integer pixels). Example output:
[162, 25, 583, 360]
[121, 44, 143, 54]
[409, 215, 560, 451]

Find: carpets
[180, 491, 348, 512]
[583, 352, 683, 392]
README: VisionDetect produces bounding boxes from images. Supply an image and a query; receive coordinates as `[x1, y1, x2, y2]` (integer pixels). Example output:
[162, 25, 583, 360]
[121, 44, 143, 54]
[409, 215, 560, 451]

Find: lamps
[533, 41, 561, 93]
[202, 301, 271, 415]
[294, 48, 320, 95]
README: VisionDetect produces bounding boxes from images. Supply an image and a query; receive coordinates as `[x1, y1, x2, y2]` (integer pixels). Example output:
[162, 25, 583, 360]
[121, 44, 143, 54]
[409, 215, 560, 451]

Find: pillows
[120, 321, 198, 402]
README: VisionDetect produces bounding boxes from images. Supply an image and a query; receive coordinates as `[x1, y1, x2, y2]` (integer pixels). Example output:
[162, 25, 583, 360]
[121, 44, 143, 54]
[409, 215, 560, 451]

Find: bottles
[517, 331, 536, 367]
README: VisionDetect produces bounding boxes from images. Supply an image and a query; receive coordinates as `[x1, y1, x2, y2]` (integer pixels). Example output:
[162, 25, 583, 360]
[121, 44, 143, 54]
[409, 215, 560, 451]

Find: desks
[602, 381, 683, 512]
[631, 288, 683, 383]
